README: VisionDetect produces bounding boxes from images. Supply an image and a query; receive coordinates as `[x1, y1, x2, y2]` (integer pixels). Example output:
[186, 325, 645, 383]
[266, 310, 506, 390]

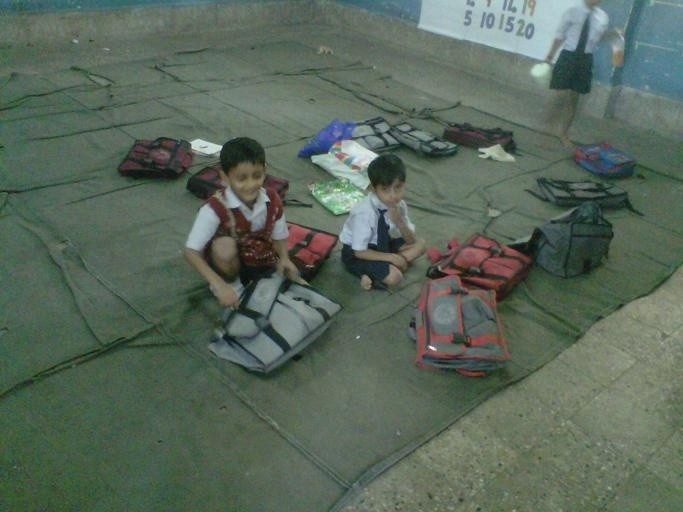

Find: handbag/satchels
[507, 201, 614, 278]
[575, 144, 634, 179]
[117, 137, 193, 180]
[207, 267, 344, 375]
[284, 221, 339, 280]
[413, 275, 508, 378]
[537, 176, 628, 207]
[428, 233, 532, 300]
[186, 162, 288, 201]
[342, 111, 520, 157]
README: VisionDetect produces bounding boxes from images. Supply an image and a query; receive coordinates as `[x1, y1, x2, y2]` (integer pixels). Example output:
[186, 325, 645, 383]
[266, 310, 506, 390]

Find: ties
[373, 207, 389, 279]
[575, 14, 590, 60]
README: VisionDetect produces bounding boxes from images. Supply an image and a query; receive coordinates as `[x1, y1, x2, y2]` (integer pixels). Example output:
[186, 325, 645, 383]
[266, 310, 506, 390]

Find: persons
[538, 0, 625, 147]
[184, 136, 299, 312]
[338, 154, 427, 291]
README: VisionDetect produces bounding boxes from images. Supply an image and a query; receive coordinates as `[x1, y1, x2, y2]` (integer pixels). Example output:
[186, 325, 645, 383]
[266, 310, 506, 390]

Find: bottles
[611, 31, 625, 66]
